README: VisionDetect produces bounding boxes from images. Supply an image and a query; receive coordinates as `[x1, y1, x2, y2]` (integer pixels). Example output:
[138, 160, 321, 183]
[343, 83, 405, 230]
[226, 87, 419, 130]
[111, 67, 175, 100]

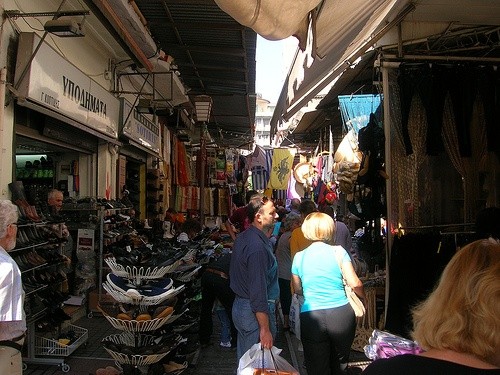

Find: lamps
[43, 15, 84, 38]
[135, 98, 154, 114]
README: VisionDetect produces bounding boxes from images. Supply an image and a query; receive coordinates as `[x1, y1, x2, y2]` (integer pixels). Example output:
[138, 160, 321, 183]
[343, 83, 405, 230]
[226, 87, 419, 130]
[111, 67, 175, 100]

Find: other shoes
[219, 341, 232, 347]
[77, 197, 135, 270]
[8, 181, 71, 332]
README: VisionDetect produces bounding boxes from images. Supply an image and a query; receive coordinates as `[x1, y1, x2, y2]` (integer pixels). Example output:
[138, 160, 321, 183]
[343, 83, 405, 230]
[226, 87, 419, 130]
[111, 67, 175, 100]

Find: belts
[0, 334, 25, 351]
[207, 268, 226, 276]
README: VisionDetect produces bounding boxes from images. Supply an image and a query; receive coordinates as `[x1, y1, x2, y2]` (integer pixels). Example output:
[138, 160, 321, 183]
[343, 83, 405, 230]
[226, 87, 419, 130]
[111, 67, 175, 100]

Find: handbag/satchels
[343, 281, 366, 318]
[288, 295, 301, 340]
[237, 342, 301, 375]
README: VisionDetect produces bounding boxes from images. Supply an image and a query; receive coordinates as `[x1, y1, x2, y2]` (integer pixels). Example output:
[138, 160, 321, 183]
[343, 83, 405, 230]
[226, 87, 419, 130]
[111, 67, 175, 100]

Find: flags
[338, 93, 385, 161]
[245, 145, 300, 201]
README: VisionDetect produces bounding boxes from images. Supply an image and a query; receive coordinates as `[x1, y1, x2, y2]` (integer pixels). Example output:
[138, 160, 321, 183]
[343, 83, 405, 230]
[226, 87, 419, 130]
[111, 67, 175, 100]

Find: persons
[127, 209, 135, 219]
[0, 200, 27, 375]
[292, 212, 369, 375]
[473, 207, 499, 238]
[199, 253, 238, 348]
[227, 190, 352, 335]
[173, 219, 203, 246]
[48, 189, 72, 309]
[229, 194, 280, 367]
[361, 238, 500, 375]
[419, 165, 464, 226]
[215, 300, 232, 347]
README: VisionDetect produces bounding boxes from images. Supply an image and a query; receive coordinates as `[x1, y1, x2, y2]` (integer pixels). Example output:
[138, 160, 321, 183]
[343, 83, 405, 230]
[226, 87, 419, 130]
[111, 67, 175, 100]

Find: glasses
[12, 223, 19, 228]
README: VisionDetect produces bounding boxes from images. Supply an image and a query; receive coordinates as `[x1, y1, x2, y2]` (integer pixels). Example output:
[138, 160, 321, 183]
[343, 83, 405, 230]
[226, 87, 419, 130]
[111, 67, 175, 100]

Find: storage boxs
[89, 293, 115, 312]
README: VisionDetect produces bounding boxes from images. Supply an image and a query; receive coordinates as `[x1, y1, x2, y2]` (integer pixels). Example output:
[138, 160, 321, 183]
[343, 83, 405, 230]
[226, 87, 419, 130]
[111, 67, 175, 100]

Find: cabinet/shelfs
[96, 247, 203, 375]
[61, 206, 129, 309]
[5, 218, 88, 372]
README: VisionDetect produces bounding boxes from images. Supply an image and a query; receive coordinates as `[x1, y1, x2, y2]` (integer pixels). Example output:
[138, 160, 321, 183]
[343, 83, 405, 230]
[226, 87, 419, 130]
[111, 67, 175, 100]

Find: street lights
[192, 93, 214, 227]
